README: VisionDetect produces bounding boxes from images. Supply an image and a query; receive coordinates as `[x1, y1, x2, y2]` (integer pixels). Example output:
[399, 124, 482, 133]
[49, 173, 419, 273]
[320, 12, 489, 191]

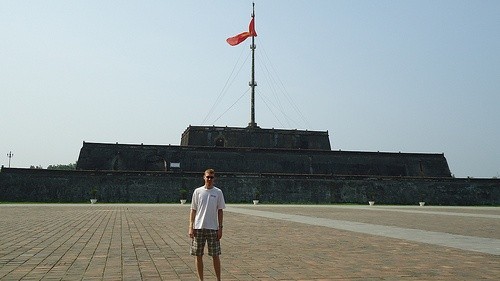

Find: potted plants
[252, 191, 260, 205]
[419, 195, 425, 206]
[368, 193, 374, 206]
[179, 190, 187, 205]
[90, 190, 98, 204]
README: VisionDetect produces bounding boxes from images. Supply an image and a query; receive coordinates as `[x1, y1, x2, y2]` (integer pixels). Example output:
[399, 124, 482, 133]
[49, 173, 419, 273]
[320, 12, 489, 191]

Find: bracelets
[218, 225, 223, 228]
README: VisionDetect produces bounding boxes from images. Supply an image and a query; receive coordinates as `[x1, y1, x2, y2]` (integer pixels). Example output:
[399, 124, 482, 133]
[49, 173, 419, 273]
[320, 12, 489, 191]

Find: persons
[189, 169, 226, 281]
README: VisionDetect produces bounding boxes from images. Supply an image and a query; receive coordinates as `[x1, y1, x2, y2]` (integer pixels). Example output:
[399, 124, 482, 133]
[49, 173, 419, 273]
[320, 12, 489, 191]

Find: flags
[226, 18, 257, 46]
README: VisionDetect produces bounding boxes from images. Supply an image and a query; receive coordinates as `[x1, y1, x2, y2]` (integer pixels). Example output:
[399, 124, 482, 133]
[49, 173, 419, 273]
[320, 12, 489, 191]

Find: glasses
[206, 176, 214, 178]
